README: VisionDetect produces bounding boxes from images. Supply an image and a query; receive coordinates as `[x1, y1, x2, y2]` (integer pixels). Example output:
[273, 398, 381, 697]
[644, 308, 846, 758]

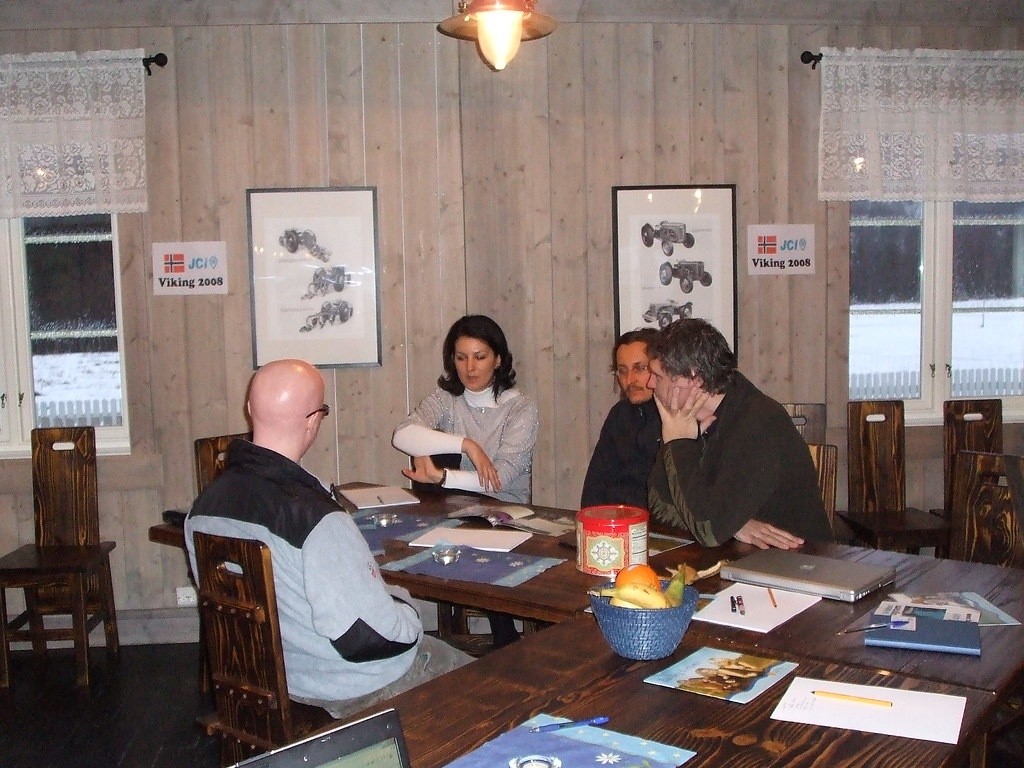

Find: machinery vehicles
[642, 298, 694, 328]
[660, 258, 713, 294]
[278, 227, 354, 333]
[641, 220, 696, 254]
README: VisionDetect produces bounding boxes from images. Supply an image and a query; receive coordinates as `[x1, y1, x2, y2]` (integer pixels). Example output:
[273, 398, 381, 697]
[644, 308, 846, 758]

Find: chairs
[1, 424, 119, 689]
[191, 399, 1024, 752]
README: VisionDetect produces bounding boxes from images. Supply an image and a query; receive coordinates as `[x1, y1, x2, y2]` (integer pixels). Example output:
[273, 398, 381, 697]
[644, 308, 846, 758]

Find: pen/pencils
[377, 496, 385, 506]
[731, 596, 736, 613]
[811, 689, 893, 707]
[766, 586, 777, 608]
[531, 715, 611, 732]
[834, 621, 910, 637]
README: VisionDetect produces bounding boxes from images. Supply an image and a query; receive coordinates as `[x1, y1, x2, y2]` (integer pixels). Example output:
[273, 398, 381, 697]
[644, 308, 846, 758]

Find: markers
[736, 595, 745, 615]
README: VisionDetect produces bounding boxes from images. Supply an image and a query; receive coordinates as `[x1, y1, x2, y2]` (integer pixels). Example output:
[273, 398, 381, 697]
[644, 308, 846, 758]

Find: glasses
[306, 404, 330, 415]
[615, 365, 647, 376]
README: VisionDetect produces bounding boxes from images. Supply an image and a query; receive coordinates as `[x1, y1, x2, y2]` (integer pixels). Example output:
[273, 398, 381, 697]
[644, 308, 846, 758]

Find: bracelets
[435, 466, 447, 488]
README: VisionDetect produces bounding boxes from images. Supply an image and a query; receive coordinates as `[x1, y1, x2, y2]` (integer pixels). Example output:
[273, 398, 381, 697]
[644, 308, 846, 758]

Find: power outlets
[176, 586, 197, 607]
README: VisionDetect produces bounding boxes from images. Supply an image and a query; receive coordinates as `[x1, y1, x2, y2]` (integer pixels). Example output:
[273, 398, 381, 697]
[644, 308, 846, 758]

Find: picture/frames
[613, 184, 739, 363]
[247, 185, 384, 373]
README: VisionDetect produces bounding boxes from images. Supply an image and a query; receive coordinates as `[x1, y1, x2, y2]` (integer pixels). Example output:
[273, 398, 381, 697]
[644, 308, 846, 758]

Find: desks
[149, 476, 1024, 768]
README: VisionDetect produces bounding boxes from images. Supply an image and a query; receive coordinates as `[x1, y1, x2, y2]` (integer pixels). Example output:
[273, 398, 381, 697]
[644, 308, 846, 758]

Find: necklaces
[463, 392, 493, 414]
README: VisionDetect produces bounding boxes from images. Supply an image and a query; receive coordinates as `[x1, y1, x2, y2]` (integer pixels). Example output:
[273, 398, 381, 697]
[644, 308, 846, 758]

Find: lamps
[439, 0, 558, 71]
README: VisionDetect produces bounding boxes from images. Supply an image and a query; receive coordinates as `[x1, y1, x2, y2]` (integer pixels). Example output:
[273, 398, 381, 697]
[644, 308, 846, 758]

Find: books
[408, 502, 578, 552]
[643, 580, 823, 707]
[437, 714, 698, 768]
[864, 591, 1023, 657]
[336, 485, 419, 513]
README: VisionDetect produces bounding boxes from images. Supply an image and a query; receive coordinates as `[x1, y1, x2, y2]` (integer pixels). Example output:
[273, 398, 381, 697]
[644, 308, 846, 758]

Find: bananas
[599, 584, 673, 609]
[665, 563, 685, 605]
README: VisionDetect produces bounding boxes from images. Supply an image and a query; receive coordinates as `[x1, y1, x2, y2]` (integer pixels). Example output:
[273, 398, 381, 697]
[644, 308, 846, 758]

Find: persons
[182, 358, 481, 722]
[391, 316, 539, 649]
[645, 317, 839, 550]
[581, 328, 663, 510]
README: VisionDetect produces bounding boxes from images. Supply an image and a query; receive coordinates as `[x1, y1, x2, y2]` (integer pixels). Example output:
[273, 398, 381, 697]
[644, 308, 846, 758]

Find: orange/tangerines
[615, 563, 661, 590]
